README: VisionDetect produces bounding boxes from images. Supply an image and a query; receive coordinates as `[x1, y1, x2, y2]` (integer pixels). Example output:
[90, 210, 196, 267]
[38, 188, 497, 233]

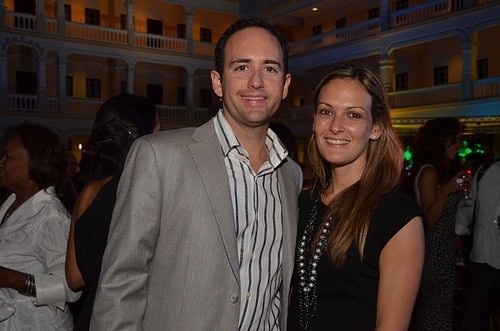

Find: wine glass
[460, 174, 473, 200]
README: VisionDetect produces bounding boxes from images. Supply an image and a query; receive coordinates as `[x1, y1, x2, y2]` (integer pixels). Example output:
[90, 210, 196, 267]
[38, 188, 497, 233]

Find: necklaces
[297, 175, 341, 331]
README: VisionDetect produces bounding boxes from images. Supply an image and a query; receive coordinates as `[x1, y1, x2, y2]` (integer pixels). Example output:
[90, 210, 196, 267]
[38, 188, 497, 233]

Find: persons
[287, 64, 424, 331]
[0, 119, 83, 331]
[59, 153, 84, 214]
[65, 95, 157, 331]
[89, 19, 303, 331]
[400, 117, 500, 331]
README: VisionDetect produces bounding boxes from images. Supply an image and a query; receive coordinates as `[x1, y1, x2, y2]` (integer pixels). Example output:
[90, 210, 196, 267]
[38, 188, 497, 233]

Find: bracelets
[18, 273, 36, 297]
